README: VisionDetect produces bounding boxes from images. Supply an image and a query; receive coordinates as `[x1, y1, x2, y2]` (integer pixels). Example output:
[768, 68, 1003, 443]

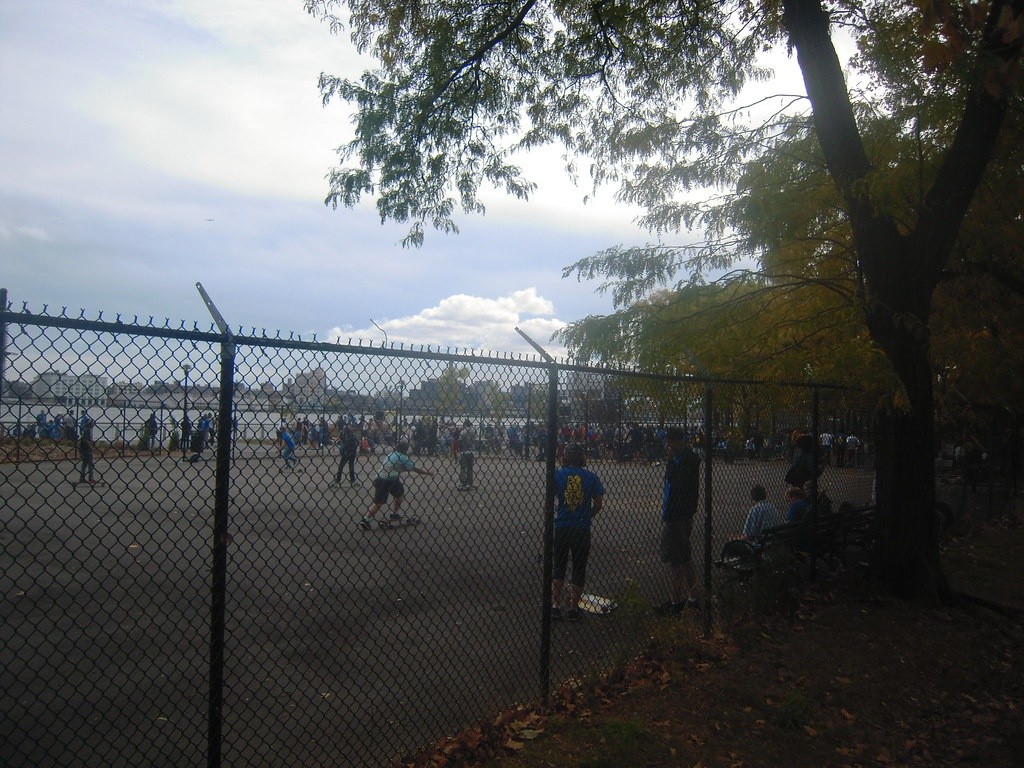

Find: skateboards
[457, 485, 477, 492]
[71, 480, 105, 488]
[378, 516, 420, 530]
[331, 482, 359, 489]
[570, 592, 618, 614]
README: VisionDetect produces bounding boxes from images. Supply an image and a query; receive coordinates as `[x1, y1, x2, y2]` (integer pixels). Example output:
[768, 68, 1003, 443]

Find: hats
[662, 429, 683, 440]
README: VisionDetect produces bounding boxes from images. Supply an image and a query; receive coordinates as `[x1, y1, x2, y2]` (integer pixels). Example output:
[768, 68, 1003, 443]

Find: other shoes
[687, 597, 698, 607]
[551, 605, 562, 618]
[359, 516, 371, 530]
[566, 609, 578, 621]
[390, 510, 402, 518]
[652, 595, 685, 610]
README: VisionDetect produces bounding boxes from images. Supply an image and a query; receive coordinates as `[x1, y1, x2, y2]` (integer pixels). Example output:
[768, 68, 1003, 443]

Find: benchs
[710, 505, 877, 603]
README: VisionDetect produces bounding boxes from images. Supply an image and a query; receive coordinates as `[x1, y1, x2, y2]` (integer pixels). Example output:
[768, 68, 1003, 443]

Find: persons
[360, 443, 435, 531]
[1, 406, 978, 568]
[651, 426, 702, 616]
[550, 443, 606, 620]
